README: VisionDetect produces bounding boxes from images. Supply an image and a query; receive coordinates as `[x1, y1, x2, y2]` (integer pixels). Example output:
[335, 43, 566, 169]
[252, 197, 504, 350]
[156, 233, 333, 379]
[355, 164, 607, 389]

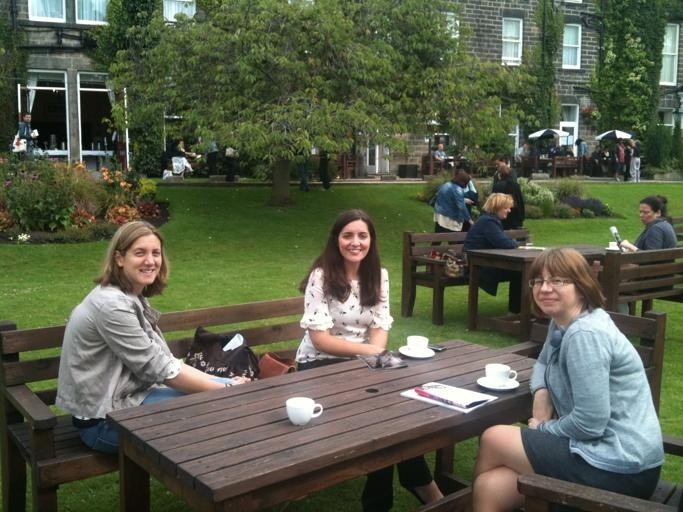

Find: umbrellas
[594, 129, 635, 143]
[527, 126, 569, 140]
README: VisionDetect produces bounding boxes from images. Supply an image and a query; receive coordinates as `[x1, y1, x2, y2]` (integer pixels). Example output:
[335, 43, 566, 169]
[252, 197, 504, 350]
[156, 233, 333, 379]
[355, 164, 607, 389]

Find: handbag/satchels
[184, 326, 259, 377]
[257, 352, 297, 379]
[427, 249, 465, 278]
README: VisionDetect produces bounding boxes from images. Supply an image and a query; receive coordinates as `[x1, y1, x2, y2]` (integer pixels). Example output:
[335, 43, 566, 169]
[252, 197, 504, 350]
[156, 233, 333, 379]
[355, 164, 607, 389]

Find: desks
[108, 338, 537, 512]
[466, 245, 611, 328]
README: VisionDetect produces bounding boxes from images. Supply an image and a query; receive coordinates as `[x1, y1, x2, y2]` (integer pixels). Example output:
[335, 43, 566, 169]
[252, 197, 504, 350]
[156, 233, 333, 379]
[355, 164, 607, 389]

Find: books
[401, 378, 498, 416]
[608, 224, 632, 254]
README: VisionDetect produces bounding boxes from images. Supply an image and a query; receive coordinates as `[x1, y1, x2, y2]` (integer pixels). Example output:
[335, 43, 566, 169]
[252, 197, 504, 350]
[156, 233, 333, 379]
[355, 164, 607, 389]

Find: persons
[12, 113, 34, 158]
[169, 133, 195, 178]
[470, 246, 665, 511]
[293, 208, 446, 512]
[460, 190, 523, 320]
[619, 195, 674, 294]
[224, 132, 242, 183]
[426, 168, 473, 246]
[295, 139, 311, 193]
[200, 131, 221, 176]
[433, 143, 447, 171]
[513, 139, 641, 183]
[488, 153, 523, 230]
[317, 142, 337, 191]
[52, 219, 252, 453]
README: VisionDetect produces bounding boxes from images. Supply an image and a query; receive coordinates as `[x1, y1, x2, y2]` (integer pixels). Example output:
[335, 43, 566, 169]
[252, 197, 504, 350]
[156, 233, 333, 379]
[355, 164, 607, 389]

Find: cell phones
[427, 344, 447, 352]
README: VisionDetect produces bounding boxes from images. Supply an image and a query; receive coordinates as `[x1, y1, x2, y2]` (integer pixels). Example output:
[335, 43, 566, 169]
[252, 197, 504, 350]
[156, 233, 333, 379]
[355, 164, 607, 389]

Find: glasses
[528, 276, 574, 288]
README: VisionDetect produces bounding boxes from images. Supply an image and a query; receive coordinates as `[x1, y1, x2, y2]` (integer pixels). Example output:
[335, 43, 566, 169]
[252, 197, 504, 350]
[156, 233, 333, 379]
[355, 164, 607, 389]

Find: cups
[484, 362, 519, 387]
[284, 396, 323, 428]
[608, 241, 617, 249]
[406, 334, 430, 355]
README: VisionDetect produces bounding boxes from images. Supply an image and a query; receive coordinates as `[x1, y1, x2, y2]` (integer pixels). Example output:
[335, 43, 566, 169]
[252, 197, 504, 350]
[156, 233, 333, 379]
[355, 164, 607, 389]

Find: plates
[473, 376, 520, 392]
[605, 247, 619, 251]
[399, 345, 435, 359]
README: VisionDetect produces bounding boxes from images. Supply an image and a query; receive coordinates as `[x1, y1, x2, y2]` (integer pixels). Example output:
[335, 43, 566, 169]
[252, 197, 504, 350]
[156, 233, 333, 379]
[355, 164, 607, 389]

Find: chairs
[517, 436, 681, 512]
[496, 310, 666, 414]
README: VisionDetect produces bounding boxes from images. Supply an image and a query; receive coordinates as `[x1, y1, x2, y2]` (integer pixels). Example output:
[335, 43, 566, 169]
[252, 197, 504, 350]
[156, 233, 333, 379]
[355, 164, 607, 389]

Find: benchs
[400, 229, 530, 324]
[422, 136, 628, 179]
[0, 295, 304, 511]
[605, 244, 682, 315]
[656, 216, 682, 241]
